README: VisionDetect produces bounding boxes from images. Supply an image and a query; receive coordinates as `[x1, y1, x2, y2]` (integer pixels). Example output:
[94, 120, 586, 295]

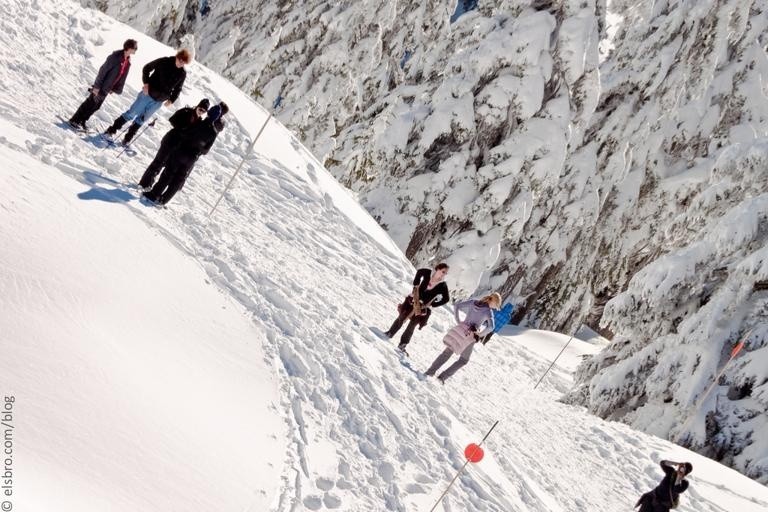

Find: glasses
[198, 108, 205, 113]
[441, 270, 448, 274]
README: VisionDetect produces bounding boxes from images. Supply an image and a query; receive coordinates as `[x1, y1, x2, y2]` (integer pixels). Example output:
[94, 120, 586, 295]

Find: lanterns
[464, 441, 485, 465]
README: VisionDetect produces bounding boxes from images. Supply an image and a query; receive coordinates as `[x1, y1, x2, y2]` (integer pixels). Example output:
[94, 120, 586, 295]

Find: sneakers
[140, 180, 165, 207]
[106, 132, 130, 148]
[70, 118, 88, 130]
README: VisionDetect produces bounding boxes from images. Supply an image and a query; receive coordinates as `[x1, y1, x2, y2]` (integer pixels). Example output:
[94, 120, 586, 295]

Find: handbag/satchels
[671, 493, 680, 510]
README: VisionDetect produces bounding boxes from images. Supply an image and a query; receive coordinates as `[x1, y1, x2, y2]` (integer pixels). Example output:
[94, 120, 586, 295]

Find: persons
[384, 263, 449, 357]
[92, 43, 193, 149]
[66, 37, 139, 132]
[141, 104, 223, 209]
[634, 460, 693, 512]
[165, 101, 230, 191]
[135, 98, 212, 191]
[424, 291, 502, 386]
[479, 301, 514, 346]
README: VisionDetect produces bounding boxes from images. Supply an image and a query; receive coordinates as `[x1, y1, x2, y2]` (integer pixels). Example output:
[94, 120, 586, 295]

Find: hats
[684, 461, 692, 476]
[207, 105, 221, 121]
[197, 99, 210, 111]
